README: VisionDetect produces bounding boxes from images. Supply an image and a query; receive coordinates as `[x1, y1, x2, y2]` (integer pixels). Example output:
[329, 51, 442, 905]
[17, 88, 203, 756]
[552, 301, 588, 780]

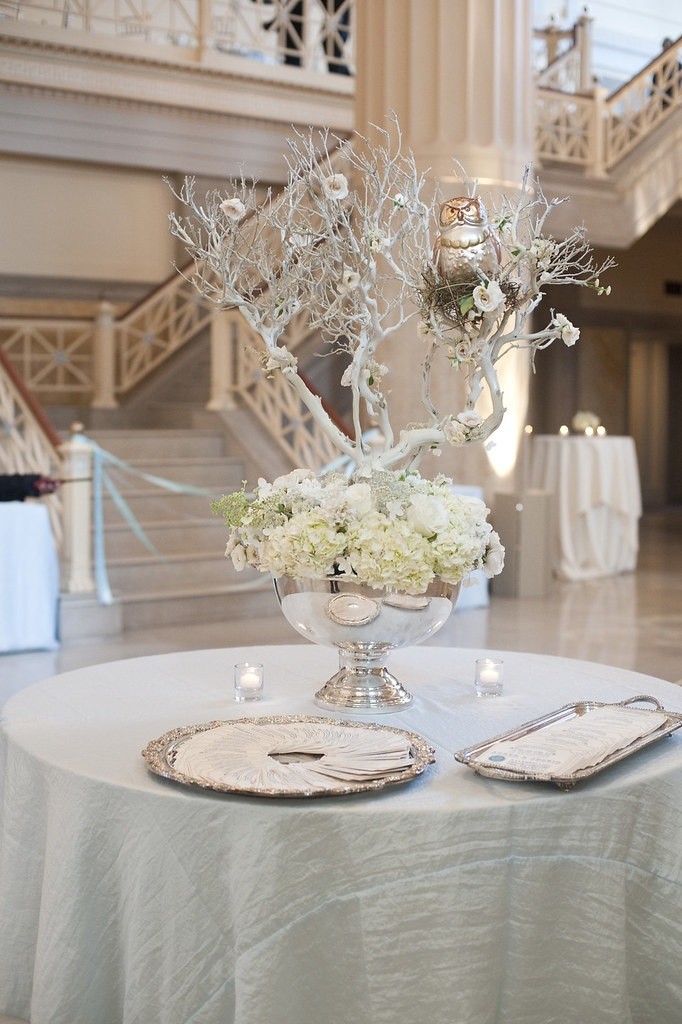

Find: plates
[454, 693, 682, 789]
[143, 715, 435, 798]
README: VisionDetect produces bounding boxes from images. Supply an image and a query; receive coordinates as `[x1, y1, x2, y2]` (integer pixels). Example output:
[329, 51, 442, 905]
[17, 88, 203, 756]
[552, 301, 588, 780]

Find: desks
[0, 500, 63, 656]
[533, 433, 642, 581]
[0, 645, 682, 1024]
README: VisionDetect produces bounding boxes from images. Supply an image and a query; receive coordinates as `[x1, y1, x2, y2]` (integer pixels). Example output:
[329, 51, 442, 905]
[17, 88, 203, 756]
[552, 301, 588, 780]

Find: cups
[234, 664, 264, 701]
[475, 659, 505, 697]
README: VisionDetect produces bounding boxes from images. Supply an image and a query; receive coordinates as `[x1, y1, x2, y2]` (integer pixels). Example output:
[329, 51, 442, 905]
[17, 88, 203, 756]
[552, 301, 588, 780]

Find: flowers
[162, 113, 618, 591]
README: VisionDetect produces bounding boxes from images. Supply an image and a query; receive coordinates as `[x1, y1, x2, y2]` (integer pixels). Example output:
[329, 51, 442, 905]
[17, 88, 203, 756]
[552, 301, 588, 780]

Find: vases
[271, 570, 461, 713]
